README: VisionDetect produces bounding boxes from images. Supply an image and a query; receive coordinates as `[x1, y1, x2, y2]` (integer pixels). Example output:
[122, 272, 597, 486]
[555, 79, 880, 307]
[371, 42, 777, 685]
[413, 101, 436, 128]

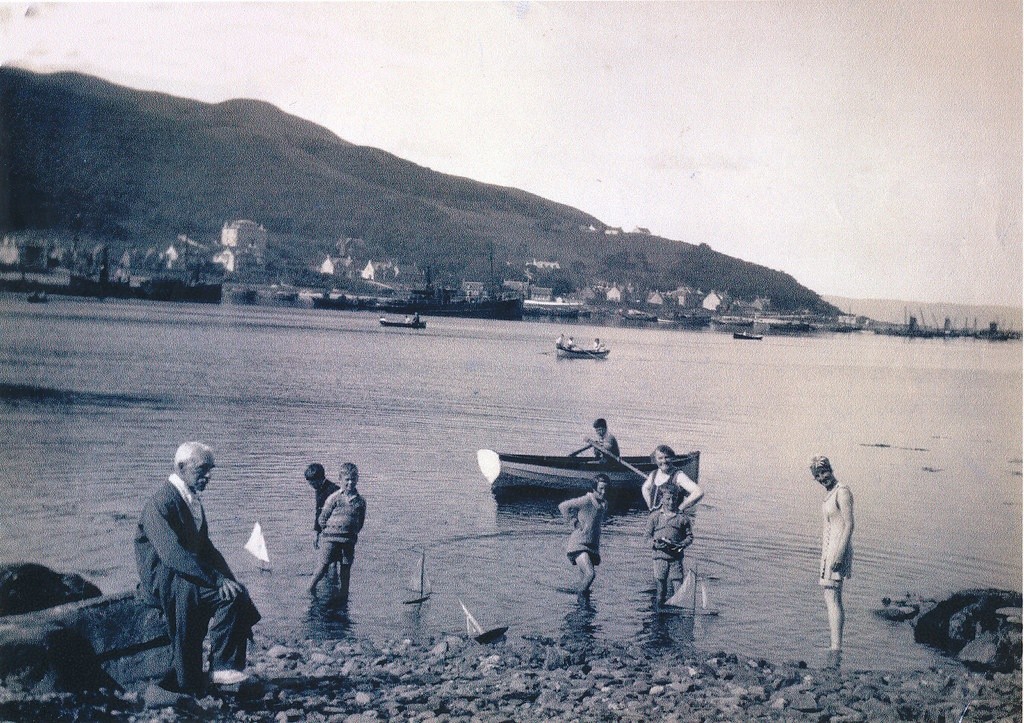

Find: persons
[134, 441, 261, 688]
[557, 474, 611, 590]
[380, 316, 385, 321]
[641, 445, 704, 513]
[556, 333, 566, 350]
[405, 316, 411, 323]
[810, 456, 854, 651]
[412, 313, 419, 322]
[567, 336, 580, 350]
[309, 463, 367, 589]
[643, 482, 694, 597]
[587, 418, 620, 464]
[595, 338, 605, 352]
[304, 462, 340, 549]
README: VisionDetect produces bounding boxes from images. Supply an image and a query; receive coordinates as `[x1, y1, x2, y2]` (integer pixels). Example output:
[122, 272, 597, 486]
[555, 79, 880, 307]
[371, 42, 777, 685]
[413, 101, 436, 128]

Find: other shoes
[209, 669, 249, 684]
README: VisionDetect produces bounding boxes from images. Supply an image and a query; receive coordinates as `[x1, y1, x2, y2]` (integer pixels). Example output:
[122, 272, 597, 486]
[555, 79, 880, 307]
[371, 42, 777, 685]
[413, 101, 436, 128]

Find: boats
[478, 448, 702, 501]
[380, 317, 427, 330]
[556, 346, 610, 360]
[733, 330, 763, 340]
